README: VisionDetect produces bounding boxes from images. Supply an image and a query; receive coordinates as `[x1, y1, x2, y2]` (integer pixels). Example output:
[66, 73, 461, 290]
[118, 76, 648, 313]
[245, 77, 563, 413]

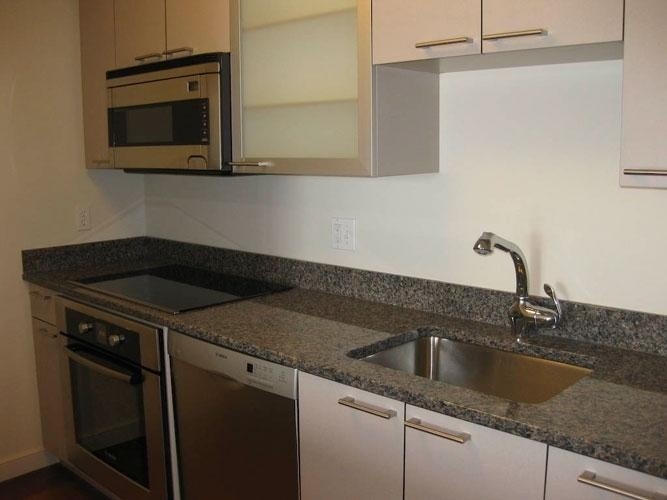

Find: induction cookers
[51, 261, 298, 375]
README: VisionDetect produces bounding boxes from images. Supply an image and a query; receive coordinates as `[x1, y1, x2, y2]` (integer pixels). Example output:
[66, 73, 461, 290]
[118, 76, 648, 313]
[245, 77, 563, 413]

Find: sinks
[355, 337, 593, 403]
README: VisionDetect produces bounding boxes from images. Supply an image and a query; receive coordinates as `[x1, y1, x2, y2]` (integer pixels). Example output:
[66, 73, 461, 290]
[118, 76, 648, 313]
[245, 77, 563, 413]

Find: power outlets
[75, 205, 90, 230]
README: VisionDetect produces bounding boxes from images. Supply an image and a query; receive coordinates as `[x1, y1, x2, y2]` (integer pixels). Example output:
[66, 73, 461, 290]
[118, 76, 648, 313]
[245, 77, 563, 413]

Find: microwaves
[104, 51, 233, 175]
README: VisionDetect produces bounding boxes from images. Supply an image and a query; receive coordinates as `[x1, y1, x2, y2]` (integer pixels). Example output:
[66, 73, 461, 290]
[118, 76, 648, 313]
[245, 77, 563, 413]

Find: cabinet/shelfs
[230, 1, 440, 177]
[372, 0, 624, 65]
[78, 0, 113, 172]
[297, 371, 547, 500]
[544, 444, 667, 500]
[618, 0, 667, 189]
[114, 0, 230, 68]
[28, 282, 73, 468]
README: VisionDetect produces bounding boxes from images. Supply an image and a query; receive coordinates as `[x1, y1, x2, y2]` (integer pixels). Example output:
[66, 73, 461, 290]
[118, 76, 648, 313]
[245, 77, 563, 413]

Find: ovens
[51, 334, 170, 499]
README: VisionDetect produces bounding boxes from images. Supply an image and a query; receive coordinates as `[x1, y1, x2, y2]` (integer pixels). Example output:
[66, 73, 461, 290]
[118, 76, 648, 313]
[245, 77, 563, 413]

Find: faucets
[473, 231, 563, 344]
